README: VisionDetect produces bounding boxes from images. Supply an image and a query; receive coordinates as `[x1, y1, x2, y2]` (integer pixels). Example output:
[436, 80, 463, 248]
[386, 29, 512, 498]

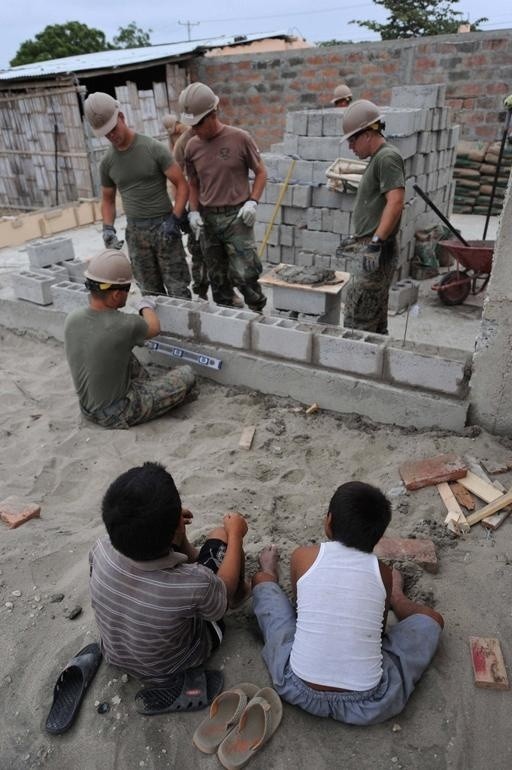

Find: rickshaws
[413, 185, 497, 306]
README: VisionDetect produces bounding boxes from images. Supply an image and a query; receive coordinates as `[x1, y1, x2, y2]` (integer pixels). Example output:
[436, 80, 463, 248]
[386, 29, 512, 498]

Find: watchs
[371, 235, 381, 244]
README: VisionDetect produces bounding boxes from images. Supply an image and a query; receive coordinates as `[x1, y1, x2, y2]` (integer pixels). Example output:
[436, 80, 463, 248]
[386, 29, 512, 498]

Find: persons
[170, 127, 245, 309]
[86, 457, 249, 681]
[329, 84, 354, 108]
[335, 99, 407, 336]
[62, 247, 202, 430]
[252, 479, 445, 727]
[160, 112, 189, 150]
[178, 81, 268, 313]
[82, 90, 192, 302]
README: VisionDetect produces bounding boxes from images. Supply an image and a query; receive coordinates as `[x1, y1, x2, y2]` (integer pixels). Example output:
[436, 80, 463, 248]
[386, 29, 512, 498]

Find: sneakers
[232, 290, 244, 308]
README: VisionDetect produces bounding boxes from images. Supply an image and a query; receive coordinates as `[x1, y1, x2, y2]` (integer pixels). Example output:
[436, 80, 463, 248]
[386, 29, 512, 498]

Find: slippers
[193, 667, 284, 770]
[136, 668, 223, 716]
[46, 643, 103, 733]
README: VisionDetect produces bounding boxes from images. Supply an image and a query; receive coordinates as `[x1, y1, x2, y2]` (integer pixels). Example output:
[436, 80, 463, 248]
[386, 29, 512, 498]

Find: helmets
[332, 85, 351, 103]
[84, 92, 120, 137]
[163, 115, 177, 135]
[178, 82, 219, 125]
[83, 248, 133, 285]
[339, 99, 383, 141]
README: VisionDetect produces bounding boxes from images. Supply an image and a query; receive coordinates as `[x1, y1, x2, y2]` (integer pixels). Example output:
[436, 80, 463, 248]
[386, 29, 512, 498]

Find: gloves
[102, 225, 124, 249]
[135, 300, 156, 315]
[159, 215, 180, 248]
[188, 211, 204, 241]
[363, 243, 382, 273]
[237, 200, 258, 227]
